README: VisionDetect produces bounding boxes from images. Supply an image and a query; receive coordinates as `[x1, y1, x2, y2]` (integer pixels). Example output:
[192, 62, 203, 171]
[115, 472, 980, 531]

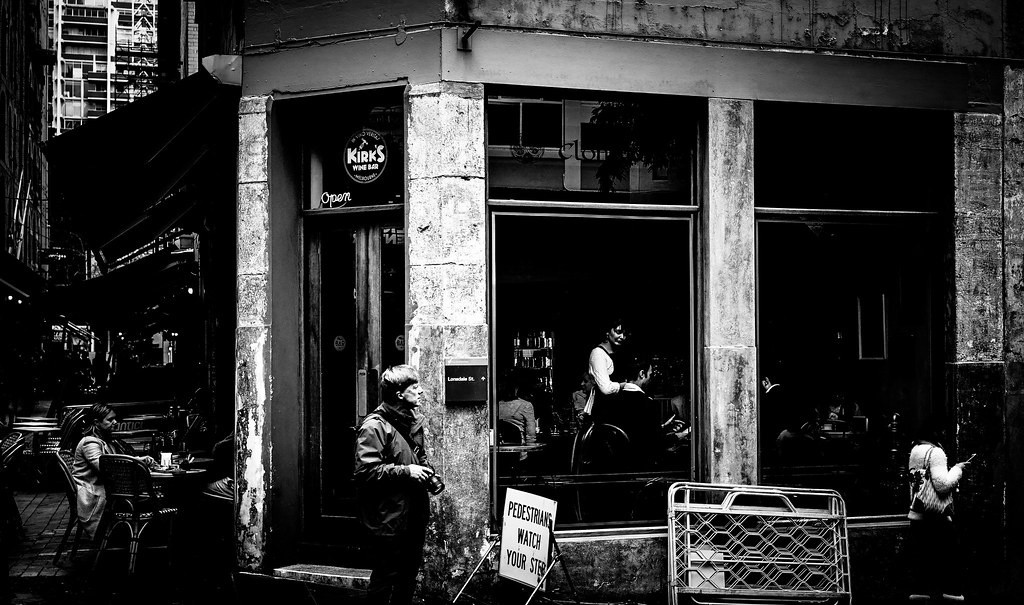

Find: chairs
[489, 400, 858, 524]
[0, 406, 235, 579]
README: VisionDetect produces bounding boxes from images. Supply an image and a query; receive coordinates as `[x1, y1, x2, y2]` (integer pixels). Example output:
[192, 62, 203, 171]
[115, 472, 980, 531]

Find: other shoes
[942, 592, 965, 601]
[910, 594, 930, 601]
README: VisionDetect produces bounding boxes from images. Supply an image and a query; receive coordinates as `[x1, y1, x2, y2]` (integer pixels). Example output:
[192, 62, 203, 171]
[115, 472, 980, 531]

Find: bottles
[511, 329, 554, 371]
[548, 423, 561, 435]
[147, 429, 181, 470]
[536, 375, 554, 395]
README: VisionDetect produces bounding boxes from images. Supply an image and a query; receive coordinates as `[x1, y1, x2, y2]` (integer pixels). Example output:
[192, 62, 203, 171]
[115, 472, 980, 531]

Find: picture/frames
[857, 293, 887, 360]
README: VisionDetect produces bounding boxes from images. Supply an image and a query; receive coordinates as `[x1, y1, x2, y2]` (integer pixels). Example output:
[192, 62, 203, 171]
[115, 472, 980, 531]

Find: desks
[490, 428, 579, 489]
[13, 414, 215, 506]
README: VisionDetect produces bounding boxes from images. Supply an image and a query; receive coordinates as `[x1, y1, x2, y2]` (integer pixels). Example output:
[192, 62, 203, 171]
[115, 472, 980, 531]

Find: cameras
[420, 464, 445, 495]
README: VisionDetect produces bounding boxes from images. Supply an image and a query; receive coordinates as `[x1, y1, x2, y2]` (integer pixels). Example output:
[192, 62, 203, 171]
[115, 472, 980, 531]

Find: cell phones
[965, 452, 977, 462]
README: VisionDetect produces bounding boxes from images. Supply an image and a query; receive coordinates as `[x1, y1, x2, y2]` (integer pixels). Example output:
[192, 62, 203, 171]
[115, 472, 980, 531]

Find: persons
[498, 368, 541, 464]
[761, 370, 809, 466]
[73, 402, 154, 542]
[908, 420, 971, 600]
[212, 402, 235, 505]
[354, 365, 444, 604]
[581, 373, 595, 396]
[583, 320, 684, 474]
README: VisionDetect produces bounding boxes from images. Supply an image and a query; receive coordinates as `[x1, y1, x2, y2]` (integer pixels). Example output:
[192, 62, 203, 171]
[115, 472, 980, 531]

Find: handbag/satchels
[909, 446, 956, 516]
